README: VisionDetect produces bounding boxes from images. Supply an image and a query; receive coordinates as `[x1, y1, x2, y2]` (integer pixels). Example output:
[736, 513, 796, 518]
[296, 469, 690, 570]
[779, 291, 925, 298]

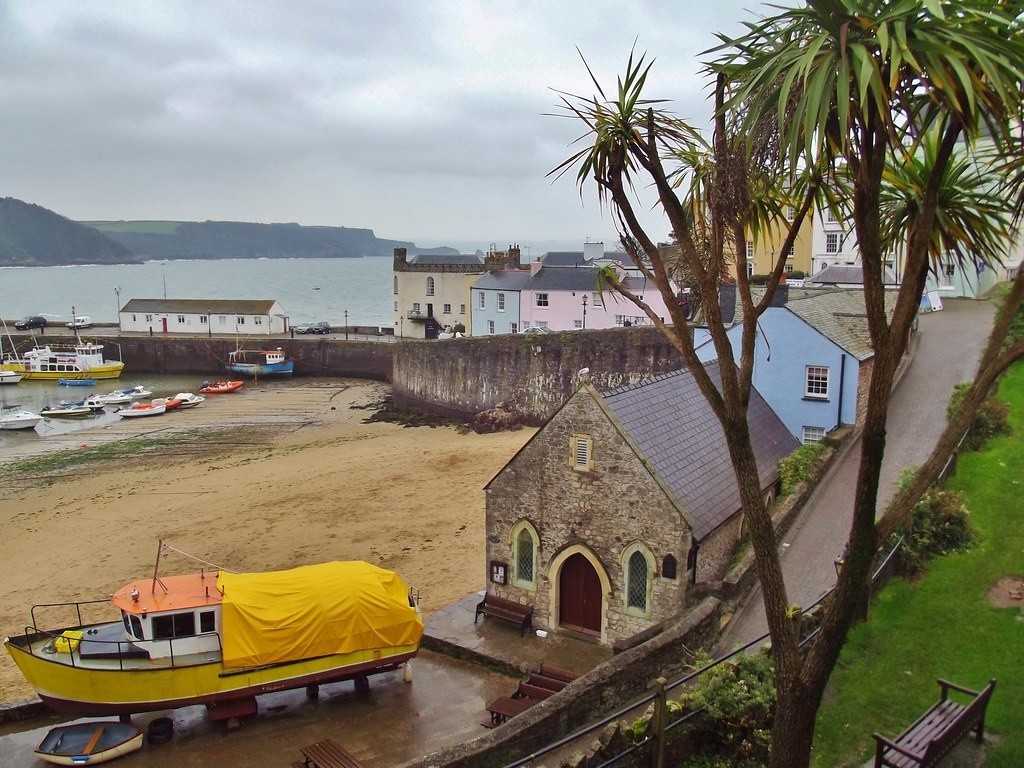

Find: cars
[295, 321, 315, 334]
[67, 315, 93, 330]
[14, 315, 48, 330]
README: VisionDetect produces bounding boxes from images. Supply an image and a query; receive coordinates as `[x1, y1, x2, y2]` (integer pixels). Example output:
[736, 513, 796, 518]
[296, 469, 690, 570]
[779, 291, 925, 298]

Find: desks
[300, 738, 364, 768]
[485, 696, 534, 726]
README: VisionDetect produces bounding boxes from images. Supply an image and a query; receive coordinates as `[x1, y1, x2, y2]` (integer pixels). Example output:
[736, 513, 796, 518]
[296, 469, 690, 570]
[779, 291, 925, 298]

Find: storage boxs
[56, 630, 83, 653]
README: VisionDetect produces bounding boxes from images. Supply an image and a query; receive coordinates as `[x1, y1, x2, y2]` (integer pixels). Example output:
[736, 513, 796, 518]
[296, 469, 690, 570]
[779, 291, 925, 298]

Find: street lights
[206, 308, 212, 337]
[454, 319, 458, 338]
[400, 315, 404, 341]
[344, 309, 349, 339]
[72, 306, 77, 335]
[580, 294, 589, 330]
[114, 285, 122, 330]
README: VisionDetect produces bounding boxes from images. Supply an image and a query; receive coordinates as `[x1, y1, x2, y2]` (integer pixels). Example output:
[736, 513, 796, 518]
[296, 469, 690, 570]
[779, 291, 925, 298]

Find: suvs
[312, 321, 332, 334]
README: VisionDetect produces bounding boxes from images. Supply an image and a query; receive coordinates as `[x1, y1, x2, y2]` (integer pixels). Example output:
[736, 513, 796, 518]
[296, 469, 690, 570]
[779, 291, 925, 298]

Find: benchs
[291, 759, 307, 768]
[871, 677, 997, 768]
[524, 670, 568, 694]
[474, 591, 533, 637]
[480, 720, 498, 728]
[538, 660, 581, 683]
[82, 728, 104, 754]
[511, 680, 557, 704]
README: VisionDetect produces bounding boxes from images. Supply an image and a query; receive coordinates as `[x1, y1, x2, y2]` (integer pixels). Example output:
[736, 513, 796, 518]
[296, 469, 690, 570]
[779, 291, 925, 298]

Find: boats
[34, 719, 145, 768]
[0, 370, 23, 383]
[39, 401, 92, 416]
[0, 402, 45, 431]
[197, 380, 245, 393]
[79, 395, 107, 411]
[58, 376, 97, 386]
[128, 398, 182, 411]
[224, 347, 295, 376]
[165, 391, 206, 409]
[112, 400, 166, 418]
[0, 316, 126, 381]
[1, 538, 425, 726]
[105, 385, 152, 404]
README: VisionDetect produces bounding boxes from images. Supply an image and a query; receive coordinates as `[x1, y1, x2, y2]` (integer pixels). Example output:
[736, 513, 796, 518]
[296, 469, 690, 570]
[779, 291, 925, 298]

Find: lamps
[566, 521, 583, 542]
